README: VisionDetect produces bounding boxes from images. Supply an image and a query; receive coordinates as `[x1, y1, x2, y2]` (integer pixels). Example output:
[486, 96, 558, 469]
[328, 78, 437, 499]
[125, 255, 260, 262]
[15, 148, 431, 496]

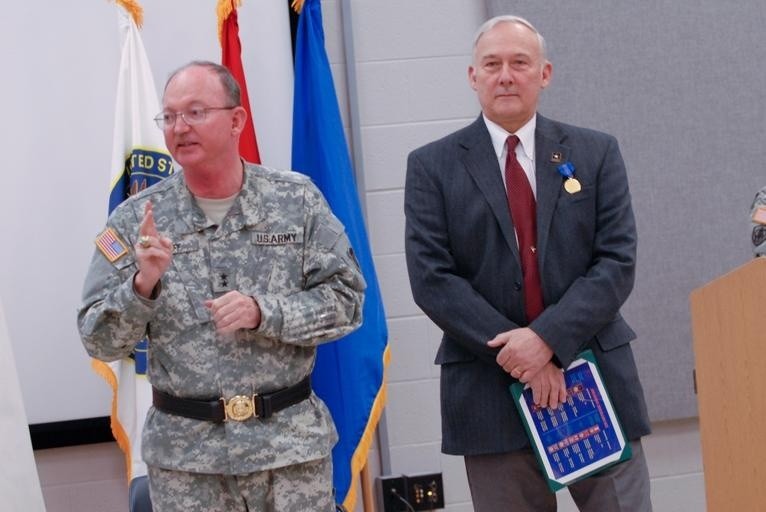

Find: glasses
[153, 107, 236, 132]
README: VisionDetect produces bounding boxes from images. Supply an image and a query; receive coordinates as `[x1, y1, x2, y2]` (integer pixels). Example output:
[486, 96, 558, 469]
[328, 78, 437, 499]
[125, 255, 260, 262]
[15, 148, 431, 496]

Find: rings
[139, 235, 149, 245]
[513, 368, 522, 375]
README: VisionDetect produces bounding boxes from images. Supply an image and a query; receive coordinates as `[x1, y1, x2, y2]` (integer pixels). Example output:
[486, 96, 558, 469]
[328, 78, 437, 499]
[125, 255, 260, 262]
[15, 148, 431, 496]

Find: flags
[216, 0, 261, 165]
[291, 1, 391, 512]
[92, 1, 176, 512]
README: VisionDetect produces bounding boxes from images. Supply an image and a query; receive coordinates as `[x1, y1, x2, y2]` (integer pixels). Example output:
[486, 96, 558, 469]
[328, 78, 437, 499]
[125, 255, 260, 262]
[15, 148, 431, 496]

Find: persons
[406, 15, 652, 512]
[78, 61, 366, 512]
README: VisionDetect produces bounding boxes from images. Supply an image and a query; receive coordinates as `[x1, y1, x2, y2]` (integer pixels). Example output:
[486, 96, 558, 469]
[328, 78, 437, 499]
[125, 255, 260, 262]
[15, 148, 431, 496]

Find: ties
[504, 135, 544, 323]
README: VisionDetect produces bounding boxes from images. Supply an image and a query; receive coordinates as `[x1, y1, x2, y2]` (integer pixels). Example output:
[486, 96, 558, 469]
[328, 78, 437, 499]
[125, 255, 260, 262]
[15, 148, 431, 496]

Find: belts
[152, 376, 311, 424]
